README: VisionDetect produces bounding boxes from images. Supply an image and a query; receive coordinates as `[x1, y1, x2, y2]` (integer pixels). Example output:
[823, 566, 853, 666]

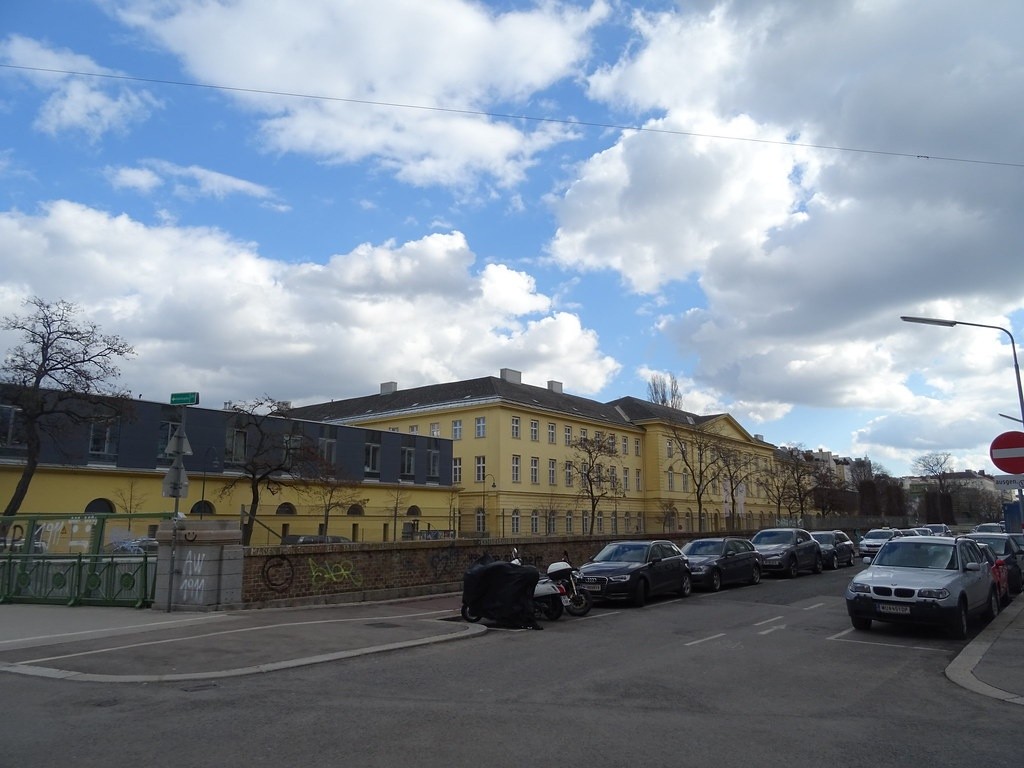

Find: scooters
[510, 547, 572, 621]
[462, 546, 540, 627]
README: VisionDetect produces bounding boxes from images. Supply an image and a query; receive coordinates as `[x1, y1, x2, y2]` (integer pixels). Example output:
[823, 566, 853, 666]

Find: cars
[975, 540, 1010, 609]
[956, 532, 1024, 593]
[102, 537, 158, 554]
[846, 534, 1000, 640]
[857, 520, 1024, 558]
[681, 536, 762, 591]
[809, 529, 855, 570]
[279, 534, 352, 544]
[750, 527, 823, 578]
[576, 539, 691, 607]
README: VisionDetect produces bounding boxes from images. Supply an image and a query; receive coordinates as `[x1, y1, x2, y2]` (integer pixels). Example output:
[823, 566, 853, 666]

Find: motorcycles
[558, 549, 593, 617]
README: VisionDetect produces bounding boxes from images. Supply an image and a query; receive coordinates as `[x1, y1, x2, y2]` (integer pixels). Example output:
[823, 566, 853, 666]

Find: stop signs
[989, 430, 1024, 474]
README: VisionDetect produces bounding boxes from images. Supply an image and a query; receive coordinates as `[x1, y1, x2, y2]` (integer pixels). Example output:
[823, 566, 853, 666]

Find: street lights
[900, 315, 1023, 426]
[482, 473, 497, 529]
